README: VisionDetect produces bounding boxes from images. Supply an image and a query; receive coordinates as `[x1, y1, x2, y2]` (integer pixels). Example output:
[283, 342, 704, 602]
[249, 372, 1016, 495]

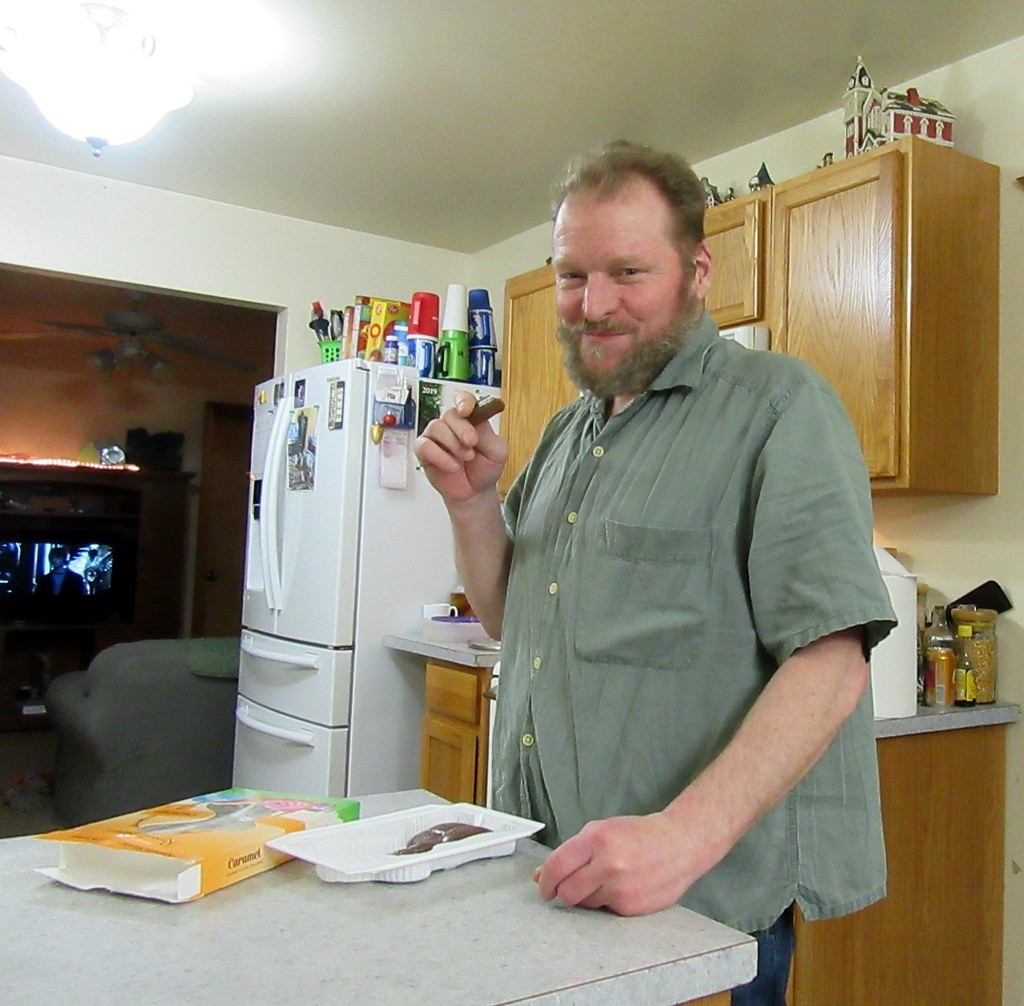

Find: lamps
[92, 336, 170, 379]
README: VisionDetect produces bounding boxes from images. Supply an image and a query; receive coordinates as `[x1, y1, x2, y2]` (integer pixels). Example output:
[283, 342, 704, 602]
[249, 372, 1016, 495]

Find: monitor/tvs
[0, 514, 138, 625]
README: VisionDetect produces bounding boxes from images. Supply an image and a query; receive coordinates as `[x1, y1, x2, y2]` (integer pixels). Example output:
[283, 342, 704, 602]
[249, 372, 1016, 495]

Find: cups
[408, 339, 436, 379]
[469, 348, 495, 386]
[437, 330, 469, 381]
[468, 289, 497, 351]
[406, 292, 439, 343]
[423, 603, 458, 619]
[442, 284, 468, 333]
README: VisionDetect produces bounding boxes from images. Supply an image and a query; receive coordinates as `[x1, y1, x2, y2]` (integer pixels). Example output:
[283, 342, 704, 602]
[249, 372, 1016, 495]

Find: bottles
[384, 335, 398, 364]
[917, 583, 930, 706]
[955, 625, 977, 707]
[923, 606, 954, 663]
[393, 320, 408, 366]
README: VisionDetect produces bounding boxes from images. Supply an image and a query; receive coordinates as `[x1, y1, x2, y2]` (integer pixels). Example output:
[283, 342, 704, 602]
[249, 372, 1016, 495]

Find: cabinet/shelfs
[498, 133, 1000, 501]
[420, 653, 1006, 1005]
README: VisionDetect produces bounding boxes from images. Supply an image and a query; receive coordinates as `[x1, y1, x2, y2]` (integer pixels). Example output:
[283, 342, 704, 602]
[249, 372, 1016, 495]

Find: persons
[83, 548, 100, 595]
[419, 135, 901, 1006]
[34, 547, 84, 594]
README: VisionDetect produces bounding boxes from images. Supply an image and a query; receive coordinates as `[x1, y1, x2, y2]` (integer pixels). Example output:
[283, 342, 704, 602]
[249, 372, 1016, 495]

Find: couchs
[46, 638, 240, 826]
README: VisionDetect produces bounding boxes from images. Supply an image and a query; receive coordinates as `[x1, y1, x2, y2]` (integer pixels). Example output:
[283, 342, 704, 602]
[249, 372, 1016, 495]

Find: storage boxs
[34, 789, 359, 903]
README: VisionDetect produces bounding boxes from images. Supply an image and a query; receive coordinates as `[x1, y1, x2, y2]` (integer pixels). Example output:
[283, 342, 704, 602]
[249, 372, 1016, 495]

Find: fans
[2, 290, 256, 376]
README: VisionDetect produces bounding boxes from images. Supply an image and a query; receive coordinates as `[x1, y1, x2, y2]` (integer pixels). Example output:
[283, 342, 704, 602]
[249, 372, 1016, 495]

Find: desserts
[393, 822, 493, 855]
[468, 393, 505, 425]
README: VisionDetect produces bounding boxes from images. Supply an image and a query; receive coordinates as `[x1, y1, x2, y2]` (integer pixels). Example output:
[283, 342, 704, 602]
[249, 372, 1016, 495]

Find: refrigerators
[231, 358, 501, 797]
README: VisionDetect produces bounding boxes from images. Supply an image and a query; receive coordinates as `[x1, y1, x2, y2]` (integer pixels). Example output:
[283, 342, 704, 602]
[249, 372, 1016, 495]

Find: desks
[0, 789, 757, 1006]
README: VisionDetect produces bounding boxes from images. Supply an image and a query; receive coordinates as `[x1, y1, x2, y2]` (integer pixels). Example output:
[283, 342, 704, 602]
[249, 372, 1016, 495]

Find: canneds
[924, 647, 955, 706]
